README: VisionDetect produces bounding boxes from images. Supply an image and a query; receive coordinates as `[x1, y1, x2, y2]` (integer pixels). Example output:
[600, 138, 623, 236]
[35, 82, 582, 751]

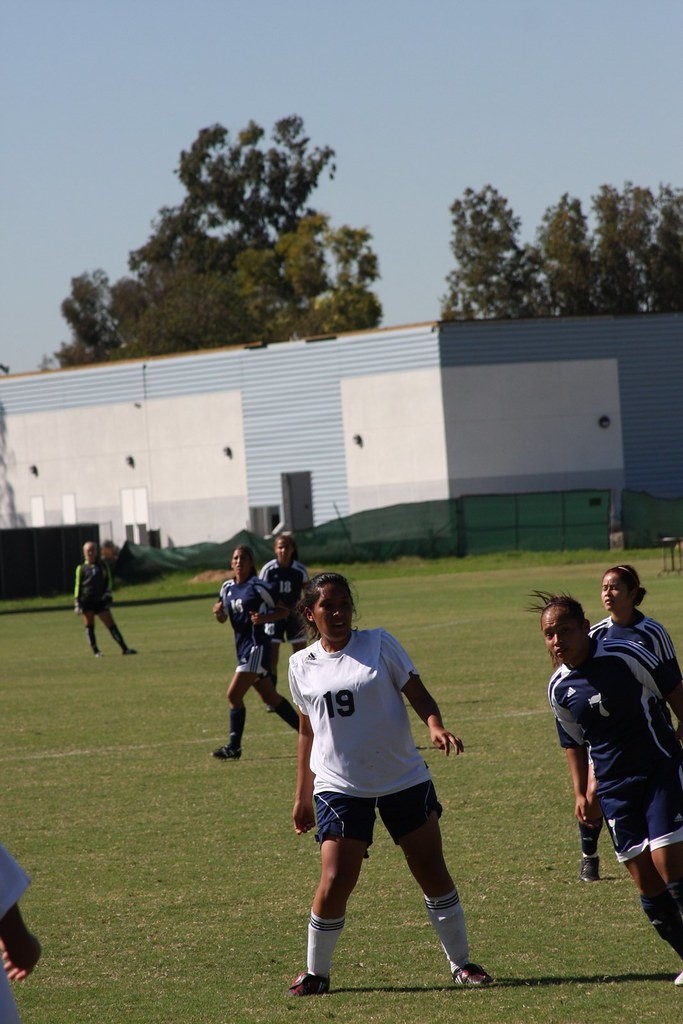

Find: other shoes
[122, 648, 137, 654]
[674, 971, 683, 986]
[95, 652, 102, 657]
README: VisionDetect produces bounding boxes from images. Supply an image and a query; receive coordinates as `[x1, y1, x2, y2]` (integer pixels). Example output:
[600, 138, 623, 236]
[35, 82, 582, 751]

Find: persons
[74, 542, 137, 657]
[287, 572, 493, 997]
[540, 563, 683, 985]
[259, 535, 310, 712]
[212, 545, 300, 761]
[0, 846, 43, 1024]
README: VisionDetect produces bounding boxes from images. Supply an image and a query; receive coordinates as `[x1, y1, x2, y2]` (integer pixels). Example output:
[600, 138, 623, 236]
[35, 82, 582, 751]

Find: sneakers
[289, 972, 330, 997]
[580, 856, 599, 883]
[452, 963, 493, 985]
[211, 745, 241, 760]
